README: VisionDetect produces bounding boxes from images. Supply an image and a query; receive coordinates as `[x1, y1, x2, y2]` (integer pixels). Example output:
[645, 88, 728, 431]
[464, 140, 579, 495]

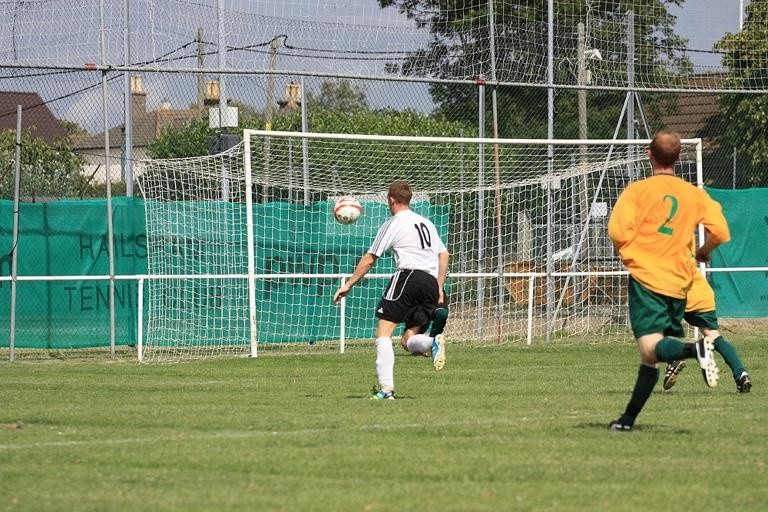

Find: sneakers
[431, 334, 446, 371]
[735, 371, 752, 393]
[369, 389, 396, 401]
[695, 335, 719, 387]
[663, 359, 686, 390]
[608, 421, 632, 431]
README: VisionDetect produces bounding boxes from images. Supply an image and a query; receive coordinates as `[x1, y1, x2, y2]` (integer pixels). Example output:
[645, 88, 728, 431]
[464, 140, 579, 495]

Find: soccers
[335, 196, 362, 224]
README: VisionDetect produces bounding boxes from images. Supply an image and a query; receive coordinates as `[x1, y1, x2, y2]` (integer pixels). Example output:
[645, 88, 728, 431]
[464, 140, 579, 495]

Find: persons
[333, 180, 449, 400]
[663, 265, 751, 395]
[608, 131, 732, 431]
